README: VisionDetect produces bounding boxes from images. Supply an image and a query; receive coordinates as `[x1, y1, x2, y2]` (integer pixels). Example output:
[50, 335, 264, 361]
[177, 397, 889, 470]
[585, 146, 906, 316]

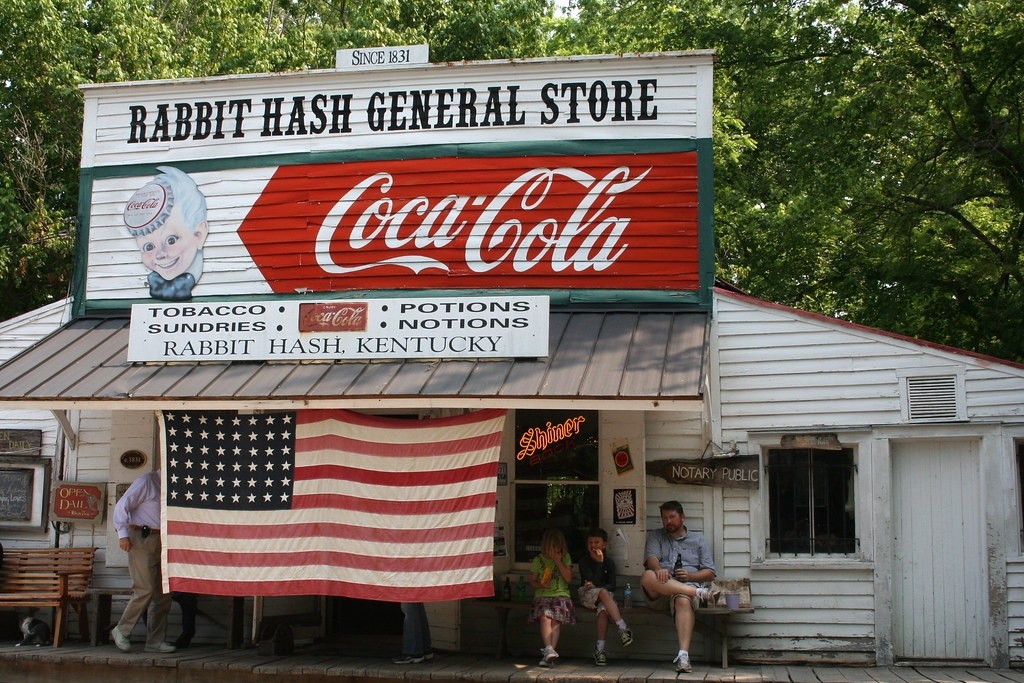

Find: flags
[155, 409, 507, 602]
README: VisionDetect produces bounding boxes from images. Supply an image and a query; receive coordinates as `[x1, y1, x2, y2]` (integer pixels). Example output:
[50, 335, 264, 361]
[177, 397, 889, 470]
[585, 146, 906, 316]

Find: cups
[725, 593, 741, 609]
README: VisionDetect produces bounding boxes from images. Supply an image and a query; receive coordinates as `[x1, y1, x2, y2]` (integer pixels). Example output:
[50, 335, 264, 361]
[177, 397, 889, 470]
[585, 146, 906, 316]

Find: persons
[529, 530, 576, 667]
[641, 500, 722, 673]
[577, 530, 634, 667]
[112, 468, 175, 653]
[391, 601, 433, 664]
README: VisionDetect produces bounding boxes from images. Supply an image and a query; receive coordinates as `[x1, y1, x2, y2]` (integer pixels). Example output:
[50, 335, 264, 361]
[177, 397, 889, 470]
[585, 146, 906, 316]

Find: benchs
[0, 547, 100, 648]
[84, 587, 242, 650]
[470, 596, 755, 669]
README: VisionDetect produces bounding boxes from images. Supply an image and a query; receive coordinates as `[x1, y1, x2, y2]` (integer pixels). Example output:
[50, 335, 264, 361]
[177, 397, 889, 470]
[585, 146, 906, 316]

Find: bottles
[516, 576, 526, 599]
[502, 577, 511, 601]
[623, 583, 633, 608]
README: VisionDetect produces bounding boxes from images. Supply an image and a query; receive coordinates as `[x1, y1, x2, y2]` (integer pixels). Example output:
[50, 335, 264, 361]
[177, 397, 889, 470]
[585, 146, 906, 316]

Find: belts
[129, 524, 160, 534]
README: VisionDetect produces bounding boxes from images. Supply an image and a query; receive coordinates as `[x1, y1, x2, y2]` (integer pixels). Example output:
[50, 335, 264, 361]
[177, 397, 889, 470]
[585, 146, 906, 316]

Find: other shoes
[175, 630, 195, 649]
[144, 641, 176, 653]
[111, 626, 130, 650]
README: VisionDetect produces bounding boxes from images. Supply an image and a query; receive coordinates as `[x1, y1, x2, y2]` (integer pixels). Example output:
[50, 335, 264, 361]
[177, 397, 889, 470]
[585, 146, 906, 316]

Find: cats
[15, 617, 51, 647]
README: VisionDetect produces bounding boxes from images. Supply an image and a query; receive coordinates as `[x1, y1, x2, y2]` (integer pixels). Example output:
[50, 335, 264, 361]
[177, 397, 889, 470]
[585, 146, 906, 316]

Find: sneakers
[539, 646, 560, 663]
[617, 628, 633, 647]
[593, 645, 607, 666]
[673, 652, 692, 672]
[400, 643, 434, 660]
[392, 653, 424, 664]
[701, 587, 721, 607]
[539, 656, 554, 667]
